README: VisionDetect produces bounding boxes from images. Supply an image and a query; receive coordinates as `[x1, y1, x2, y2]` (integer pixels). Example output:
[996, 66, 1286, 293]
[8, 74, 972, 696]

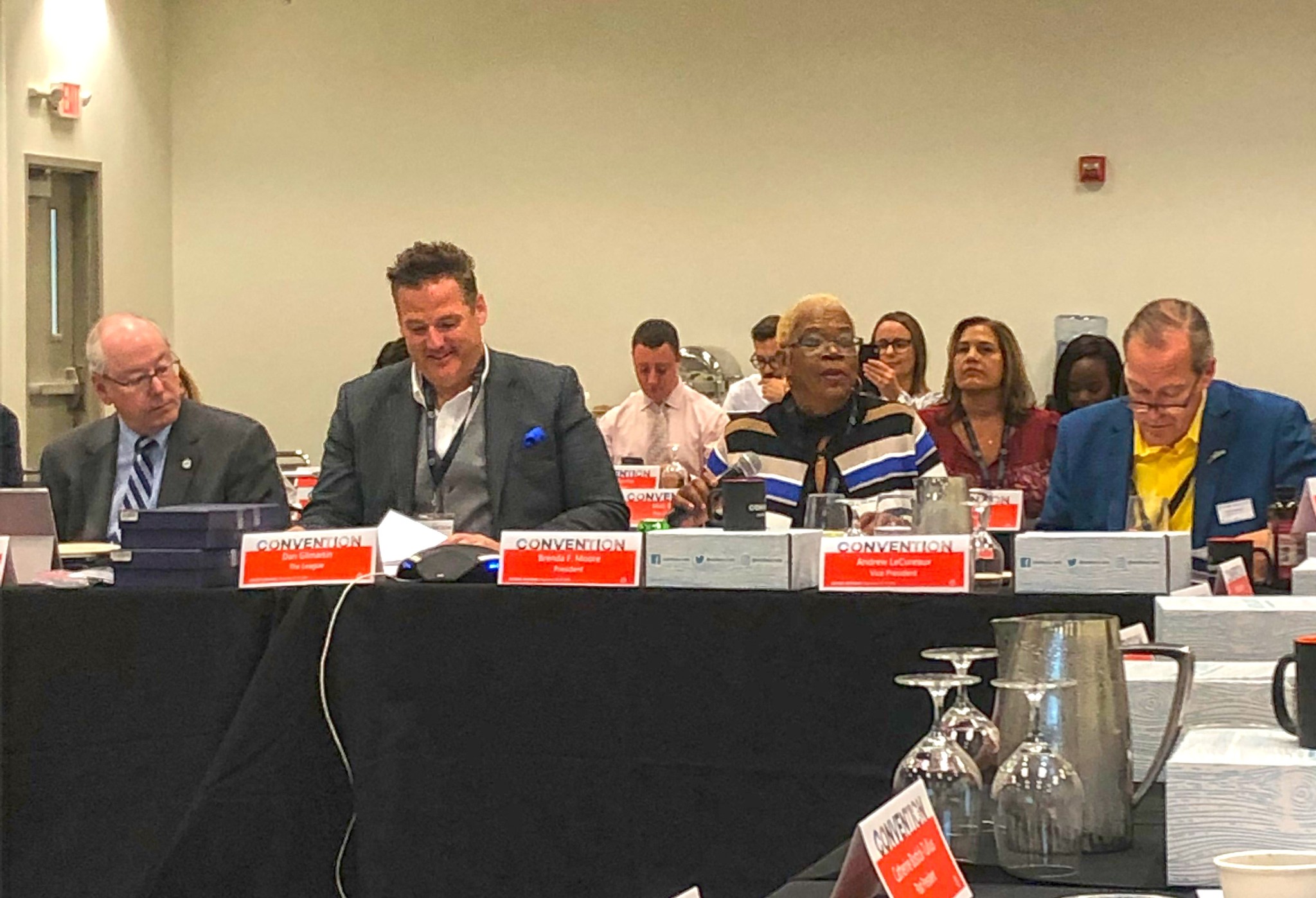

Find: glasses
[1118, 365, 1200, 415]
[102, 353, 181, 389]
[750, 352, 782, 370]
[789, 335, 864, 357]
[871, 340, 915, 354]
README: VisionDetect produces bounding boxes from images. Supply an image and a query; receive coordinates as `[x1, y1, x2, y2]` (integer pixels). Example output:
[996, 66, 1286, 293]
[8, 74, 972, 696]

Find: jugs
[913, 476, 994, 537]
[986, 616, 1192, 854]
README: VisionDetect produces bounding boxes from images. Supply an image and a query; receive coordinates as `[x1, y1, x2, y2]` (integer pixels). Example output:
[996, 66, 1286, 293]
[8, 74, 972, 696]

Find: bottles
[1053, 315, 1107, 366]
[1265, 484, 1304, 592]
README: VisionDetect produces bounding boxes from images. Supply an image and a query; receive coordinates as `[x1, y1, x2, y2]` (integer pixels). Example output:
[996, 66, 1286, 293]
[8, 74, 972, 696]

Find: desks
[0, 583, 1220, 898]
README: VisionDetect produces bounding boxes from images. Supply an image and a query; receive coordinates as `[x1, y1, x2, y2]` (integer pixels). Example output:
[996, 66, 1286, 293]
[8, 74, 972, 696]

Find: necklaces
[972, 412, 1004, 448]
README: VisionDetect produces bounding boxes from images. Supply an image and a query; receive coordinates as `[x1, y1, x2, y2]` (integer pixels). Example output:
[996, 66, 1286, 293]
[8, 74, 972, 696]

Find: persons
[1040, 334, 1129, 419]
[918, 315, 1064, 524]
[856, 308, 950, 412]
[39, 313, 291, 566]
[1035, 297, 1316, 579]
[165, 339, 201, 404]
[595, 318, 731, 487]
[286, 241, 631, 555]
[668, 292, 950, 536]
[720, 313, 792, 420]
[0, 403, 24, 489]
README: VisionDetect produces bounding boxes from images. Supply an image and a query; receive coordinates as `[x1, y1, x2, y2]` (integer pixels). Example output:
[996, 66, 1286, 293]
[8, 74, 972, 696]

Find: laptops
[0, 488, 110, 569]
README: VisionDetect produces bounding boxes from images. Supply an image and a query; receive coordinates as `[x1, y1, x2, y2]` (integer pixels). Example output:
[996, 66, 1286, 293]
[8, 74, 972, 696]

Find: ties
[109, 436, 156, 544]
[646, 405, 674, 465]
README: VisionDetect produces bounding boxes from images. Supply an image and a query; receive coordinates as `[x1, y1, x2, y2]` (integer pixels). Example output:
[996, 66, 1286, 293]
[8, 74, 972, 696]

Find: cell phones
[857, 344, 879, 365]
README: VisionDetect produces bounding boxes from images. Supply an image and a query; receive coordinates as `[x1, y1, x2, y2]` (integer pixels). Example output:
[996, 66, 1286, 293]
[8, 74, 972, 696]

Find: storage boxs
[647, 526, 824, 591]
[1013, 531, 1316, 886]
[110, 504, 291, 586]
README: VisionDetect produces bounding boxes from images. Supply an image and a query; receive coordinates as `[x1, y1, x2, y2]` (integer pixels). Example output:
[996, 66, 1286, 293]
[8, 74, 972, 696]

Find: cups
[1212, 848, 1316, 898]
[874, 493, 914, 536]
[1207, 539, 1273, 597]
[803, 493, 849, 530]
[1272, 634, 1316, 750]
[707, 477, 767, 530]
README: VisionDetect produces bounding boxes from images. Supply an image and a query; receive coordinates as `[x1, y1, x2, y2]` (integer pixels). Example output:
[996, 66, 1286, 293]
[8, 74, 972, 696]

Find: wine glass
[917, 645, 1007, 808]
[890, 671, 989, 865]
[983, 675, 1088, 876]
[965, 496, 1004, 592]
[660, 444, 686, 490]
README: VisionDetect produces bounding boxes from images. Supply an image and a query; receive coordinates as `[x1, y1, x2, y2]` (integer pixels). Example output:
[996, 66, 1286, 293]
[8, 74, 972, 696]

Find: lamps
[28, 82, 92, 120]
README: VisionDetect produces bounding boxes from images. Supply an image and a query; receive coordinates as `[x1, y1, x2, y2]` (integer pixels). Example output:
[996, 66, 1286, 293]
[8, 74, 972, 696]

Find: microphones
[663, 452, 759, 525]
